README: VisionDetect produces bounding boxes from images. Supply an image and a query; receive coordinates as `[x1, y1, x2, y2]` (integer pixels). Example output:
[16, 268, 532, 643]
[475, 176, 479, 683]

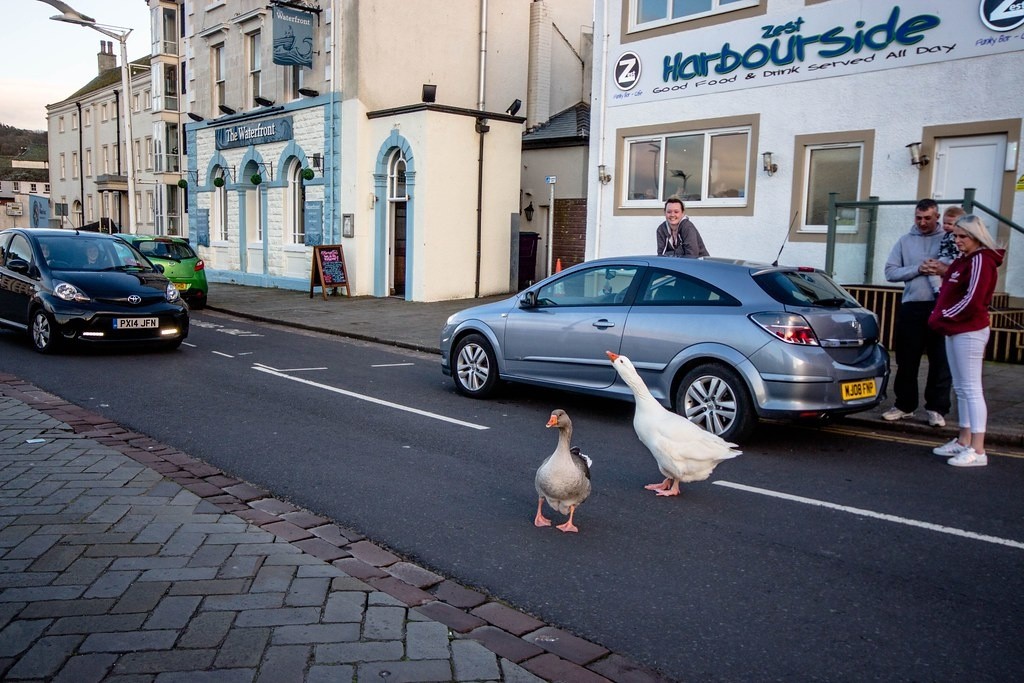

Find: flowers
[172, 147, 178, 165]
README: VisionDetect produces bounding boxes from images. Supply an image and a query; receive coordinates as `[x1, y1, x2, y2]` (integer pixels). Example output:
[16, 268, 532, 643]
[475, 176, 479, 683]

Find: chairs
[654, 277, 700, 300]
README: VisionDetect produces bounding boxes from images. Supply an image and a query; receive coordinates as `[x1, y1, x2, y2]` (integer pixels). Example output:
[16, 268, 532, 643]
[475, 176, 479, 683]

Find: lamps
[905, 142, 930, 171]
[506, 99, 522, 116]
[762, 152, 778, 176]
[218, 105, 236, 115]
[421, 84, 437, 102]
[254, 96, 275, 107]
[369, 192, 379, 209]
[524, 201, 535, 222]
[297, 88, 319, 97]
[598, 165, 612, 185]
[187, 113, 204, 122]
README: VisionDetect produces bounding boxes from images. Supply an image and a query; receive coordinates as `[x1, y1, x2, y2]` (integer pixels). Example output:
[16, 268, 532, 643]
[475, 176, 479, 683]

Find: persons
[74, 243, 113, 269]
[656, 195, 711, 299]
[881, 197, 953, 427]
[928, 216, 1007, 466]
[928, 207, 968, 303]
[34, 243, 55, 269]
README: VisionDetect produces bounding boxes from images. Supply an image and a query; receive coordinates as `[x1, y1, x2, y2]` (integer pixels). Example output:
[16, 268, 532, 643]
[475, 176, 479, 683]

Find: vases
[174, 165, 178, 172]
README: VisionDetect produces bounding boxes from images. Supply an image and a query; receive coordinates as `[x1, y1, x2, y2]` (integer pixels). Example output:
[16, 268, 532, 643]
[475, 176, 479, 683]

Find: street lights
[50, 15, 138, 236]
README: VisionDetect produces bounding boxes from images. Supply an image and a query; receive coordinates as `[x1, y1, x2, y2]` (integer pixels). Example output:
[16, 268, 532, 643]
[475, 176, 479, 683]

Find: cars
[439, 255, 891, 445]
[0, 228, 191, 354]
[97, 233, 208, 310]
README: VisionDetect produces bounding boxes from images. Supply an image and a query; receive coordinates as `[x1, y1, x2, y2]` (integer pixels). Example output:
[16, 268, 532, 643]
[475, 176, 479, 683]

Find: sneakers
[882, 407, 913, 420]
[947, 447, 987, 467]
[926, 410, 945, 426]
[932, 438, 970, 456]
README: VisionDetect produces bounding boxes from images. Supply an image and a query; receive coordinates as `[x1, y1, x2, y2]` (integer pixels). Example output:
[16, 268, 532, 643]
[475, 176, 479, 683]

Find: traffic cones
[553, 258, 566, 298]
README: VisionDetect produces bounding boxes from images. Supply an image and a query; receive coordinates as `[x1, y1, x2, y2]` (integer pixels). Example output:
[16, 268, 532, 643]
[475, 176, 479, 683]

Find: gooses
[533, 409, 594, 533]
[605, 350, 744, 498]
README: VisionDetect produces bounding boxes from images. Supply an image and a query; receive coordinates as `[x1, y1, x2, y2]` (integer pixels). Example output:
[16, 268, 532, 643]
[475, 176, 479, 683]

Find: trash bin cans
[519, 232, 540, 281]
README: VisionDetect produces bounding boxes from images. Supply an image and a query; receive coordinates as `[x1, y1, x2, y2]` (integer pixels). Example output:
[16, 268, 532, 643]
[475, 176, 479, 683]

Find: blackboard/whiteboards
[308, 244, 348, 287]
